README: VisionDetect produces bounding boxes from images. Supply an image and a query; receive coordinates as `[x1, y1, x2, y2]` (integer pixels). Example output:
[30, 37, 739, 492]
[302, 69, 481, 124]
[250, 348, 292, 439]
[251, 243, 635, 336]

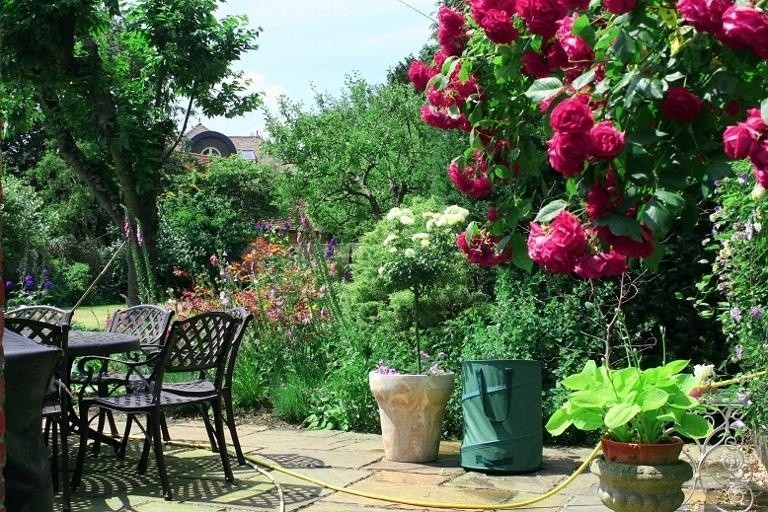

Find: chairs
[75, 309, 240, 501]
[113, 307, 253, 467]
[3, 296, 78, 494]
[2, 314, 73, 510]
[69, 305, 176, 471]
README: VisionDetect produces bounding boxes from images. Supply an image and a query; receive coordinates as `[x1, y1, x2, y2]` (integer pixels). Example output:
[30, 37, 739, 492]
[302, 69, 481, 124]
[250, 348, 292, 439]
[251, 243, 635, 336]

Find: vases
[365, 369, 458, 465]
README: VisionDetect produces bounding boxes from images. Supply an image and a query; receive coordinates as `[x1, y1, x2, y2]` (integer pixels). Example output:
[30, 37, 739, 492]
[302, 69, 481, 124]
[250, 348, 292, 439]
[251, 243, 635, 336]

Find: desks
[22, 318, 142, 454]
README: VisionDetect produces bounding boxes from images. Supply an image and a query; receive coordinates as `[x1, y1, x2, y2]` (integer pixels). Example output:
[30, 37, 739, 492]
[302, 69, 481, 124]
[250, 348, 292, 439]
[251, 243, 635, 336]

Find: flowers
[678, 356, 754, 411]
[353, 202, 485, 377]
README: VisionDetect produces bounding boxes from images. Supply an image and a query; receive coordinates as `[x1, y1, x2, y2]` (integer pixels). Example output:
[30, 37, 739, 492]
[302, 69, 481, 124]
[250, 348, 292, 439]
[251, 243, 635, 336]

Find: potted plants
[547, 356, 715, 468]
